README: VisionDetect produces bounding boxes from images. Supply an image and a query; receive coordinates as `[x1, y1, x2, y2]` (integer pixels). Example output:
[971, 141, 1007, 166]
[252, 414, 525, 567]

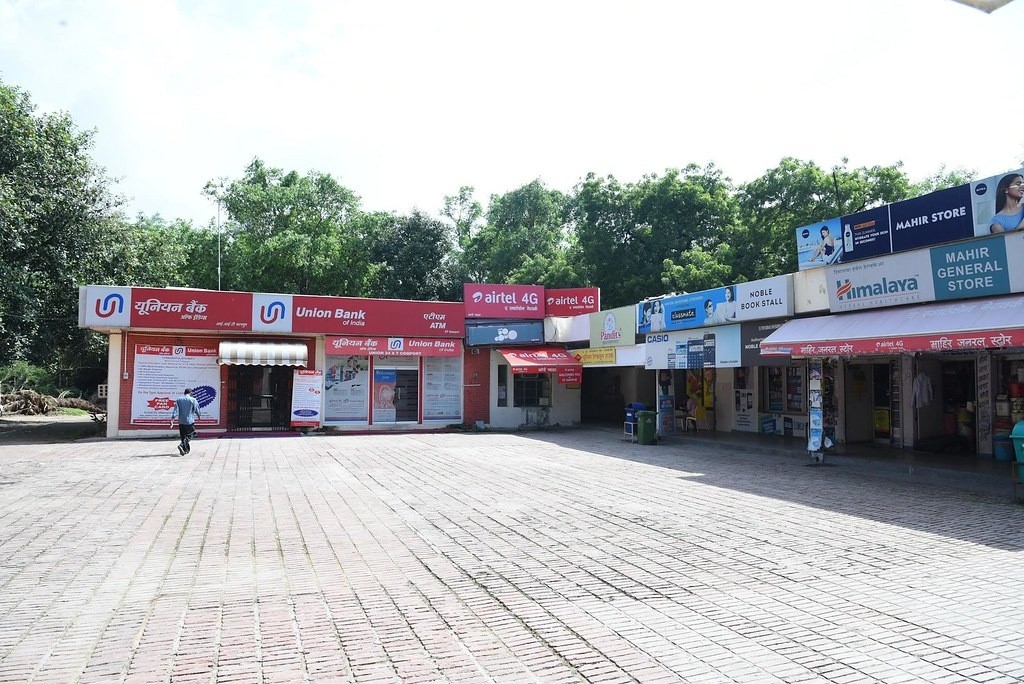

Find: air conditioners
[519, 373, 538, 379]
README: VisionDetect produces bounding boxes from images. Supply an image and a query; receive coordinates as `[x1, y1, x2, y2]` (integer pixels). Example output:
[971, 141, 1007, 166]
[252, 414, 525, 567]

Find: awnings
[760, 295, 1024, 355]
[218, 341, 308, 368]
[497, 347, 582, 384]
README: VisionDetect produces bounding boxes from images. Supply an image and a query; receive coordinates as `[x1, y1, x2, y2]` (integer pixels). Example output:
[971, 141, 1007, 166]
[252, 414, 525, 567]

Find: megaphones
[471, 349, 479, 354]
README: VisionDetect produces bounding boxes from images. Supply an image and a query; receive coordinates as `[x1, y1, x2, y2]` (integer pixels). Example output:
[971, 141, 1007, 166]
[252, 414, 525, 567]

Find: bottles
[844, 224, 853, 252]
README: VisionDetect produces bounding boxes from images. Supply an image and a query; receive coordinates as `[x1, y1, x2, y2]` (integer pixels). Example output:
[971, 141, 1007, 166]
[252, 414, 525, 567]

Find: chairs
[685, 406, 710, 432]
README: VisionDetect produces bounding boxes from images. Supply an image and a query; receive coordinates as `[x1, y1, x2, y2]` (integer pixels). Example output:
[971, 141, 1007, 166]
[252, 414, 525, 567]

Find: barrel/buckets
[993, 420, 1011, 461]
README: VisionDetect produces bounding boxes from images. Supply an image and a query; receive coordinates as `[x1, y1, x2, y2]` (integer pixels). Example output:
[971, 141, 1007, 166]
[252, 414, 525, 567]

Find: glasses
[706, 303, 713, 308]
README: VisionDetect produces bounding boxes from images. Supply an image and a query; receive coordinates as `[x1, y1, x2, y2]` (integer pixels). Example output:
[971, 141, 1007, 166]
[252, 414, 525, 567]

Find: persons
[638, 301, 666, 333]
[808, 225, 834, 262]
[658, 369, 674, 396]
[344, 359, 363, 382]
[170, 388, 201, 456]
[703, 286, 736, 325]
[988, 174, 1024, 233]
[681, 395, 697, 433]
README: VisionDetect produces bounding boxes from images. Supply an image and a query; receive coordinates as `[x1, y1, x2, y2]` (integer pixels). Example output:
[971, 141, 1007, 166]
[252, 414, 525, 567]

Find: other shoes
[178, 445, 185, 456]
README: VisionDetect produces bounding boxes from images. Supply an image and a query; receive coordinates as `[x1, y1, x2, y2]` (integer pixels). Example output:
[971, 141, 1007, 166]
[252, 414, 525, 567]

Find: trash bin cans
[635, 409, 659, 445]
[1009, 419, 1023, 480]
[625, 401, 648, 435]
